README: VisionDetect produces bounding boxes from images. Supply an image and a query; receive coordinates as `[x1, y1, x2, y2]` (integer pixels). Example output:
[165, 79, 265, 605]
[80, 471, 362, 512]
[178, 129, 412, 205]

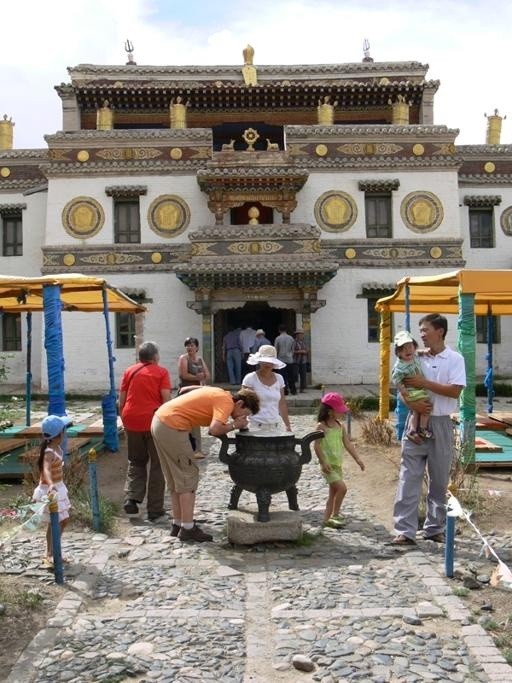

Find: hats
[41, 414, 73, 440]
[255, 329, 265, 337]
[294, 327, 305, 334]
[247, 343, 287, 370]
[393, 330, 414, 347]
[321, 392, 350, 413]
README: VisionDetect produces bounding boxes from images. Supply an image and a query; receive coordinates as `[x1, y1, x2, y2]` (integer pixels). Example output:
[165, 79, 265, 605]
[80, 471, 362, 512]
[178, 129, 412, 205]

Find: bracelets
[231, 422, 236, 430]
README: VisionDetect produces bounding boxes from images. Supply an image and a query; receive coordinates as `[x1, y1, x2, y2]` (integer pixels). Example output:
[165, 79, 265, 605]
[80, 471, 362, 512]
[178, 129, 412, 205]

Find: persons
[241, 345, 292, 432]
[151, 385, 260, 542]
[29, 414, 74, 565]
[391, 314, 467, 544]
[314, 392, 365, 528]
[391, 330, 433, 445]
[118, 341, 172, 520]
[222, 323, 309, 395]
[176, 337, 210, 458]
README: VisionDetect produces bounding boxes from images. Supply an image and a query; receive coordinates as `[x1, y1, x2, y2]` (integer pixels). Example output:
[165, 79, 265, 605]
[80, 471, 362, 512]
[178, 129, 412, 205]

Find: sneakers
[42, 557, 54, 568]
[194, 451, 205, 458]
[124, 499, 138, 513]
[321, 515, 347, 528]
[170, 521, 213, 542]
[147, 508, 166, 520]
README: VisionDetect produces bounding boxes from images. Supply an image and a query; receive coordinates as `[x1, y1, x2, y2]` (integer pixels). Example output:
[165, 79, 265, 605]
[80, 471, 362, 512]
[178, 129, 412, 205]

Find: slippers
[424, 531, 446, 543]
[391, 533, 417, 545]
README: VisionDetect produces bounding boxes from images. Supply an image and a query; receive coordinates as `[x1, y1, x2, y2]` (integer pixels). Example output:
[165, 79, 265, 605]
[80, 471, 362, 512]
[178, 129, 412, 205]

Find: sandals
[406, 430, 422, 445]
[418, 427, 435, 440]
